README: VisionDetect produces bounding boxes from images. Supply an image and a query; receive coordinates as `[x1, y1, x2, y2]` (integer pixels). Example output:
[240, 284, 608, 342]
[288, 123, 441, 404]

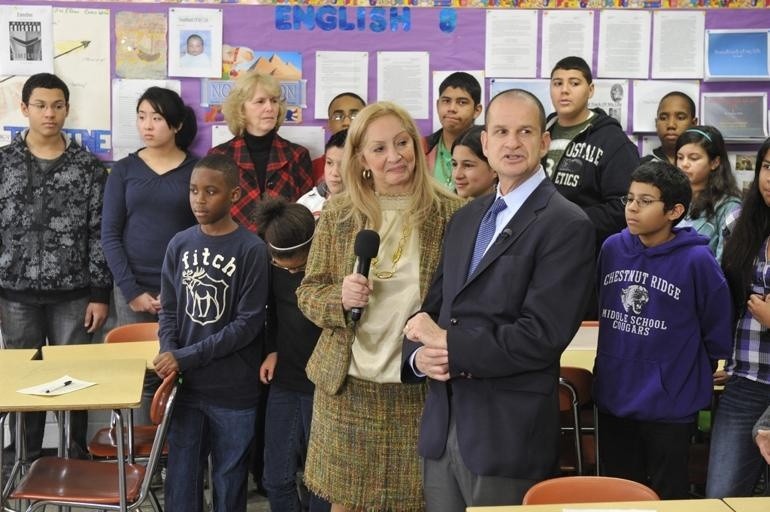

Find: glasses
[26, 101, 65, 113]
[328, 111, 358, 121]
[619, 195, 661, 207]
[271, 257, 306, 271]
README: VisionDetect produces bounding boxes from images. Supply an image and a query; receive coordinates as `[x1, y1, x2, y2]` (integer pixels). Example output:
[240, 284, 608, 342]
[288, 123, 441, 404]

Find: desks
[0, 339, 160, 512]
[559, 350, 598, 373]
[465, 498, 734, 512]
[722, 497, 770, 512]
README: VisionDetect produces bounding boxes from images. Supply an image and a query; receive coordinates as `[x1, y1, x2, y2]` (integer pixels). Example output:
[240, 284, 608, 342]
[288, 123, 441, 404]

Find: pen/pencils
[45, 380, 74, 394]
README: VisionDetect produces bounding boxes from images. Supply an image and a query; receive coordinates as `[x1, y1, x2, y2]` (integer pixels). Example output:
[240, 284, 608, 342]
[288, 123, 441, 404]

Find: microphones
[351, 230, 380, 320]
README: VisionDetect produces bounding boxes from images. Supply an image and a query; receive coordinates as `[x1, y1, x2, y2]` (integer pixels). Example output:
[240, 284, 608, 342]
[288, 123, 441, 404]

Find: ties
[467, 199, 507, 279]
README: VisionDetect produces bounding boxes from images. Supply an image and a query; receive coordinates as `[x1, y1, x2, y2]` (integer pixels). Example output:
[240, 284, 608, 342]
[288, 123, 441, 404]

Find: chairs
[523, 476, 661, 504]
[87, 322, 214, 512]
[8, 370, 178, 512]
[558, 367, 595, 475]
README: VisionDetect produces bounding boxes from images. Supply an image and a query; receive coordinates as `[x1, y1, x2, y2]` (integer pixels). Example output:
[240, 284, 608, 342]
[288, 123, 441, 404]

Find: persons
[0, 73, 109, 460]
[181, 35, 206, 62]
[102, 55, 770, 512]
